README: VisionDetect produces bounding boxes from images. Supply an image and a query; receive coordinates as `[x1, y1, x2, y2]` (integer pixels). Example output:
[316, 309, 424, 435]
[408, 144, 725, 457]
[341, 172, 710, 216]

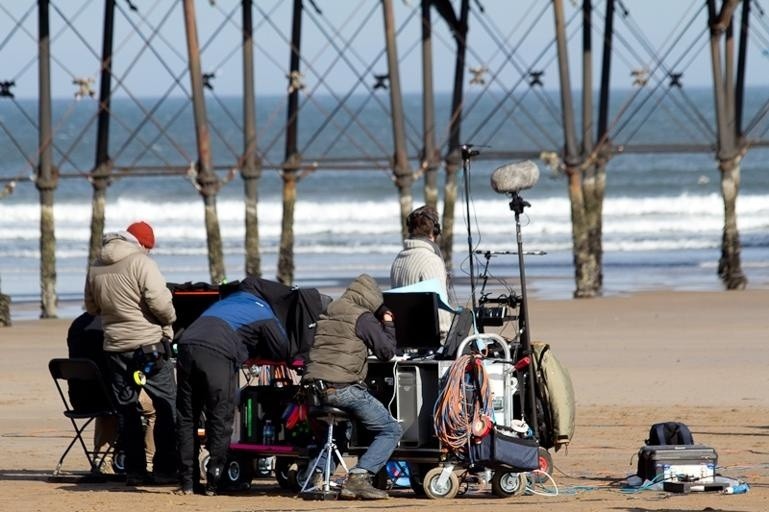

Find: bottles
[262, 419, 274, 445]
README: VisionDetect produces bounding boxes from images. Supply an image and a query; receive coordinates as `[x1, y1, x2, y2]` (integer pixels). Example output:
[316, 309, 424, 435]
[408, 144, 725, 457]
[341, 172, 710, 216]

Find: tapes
[300, 403, 308, 422]
[510, 420, 529, 433]
[281, 403, 294, 419]
[286, 404, 300, 430]
[472, 415, 492, 438]
[133, 370, 146, 386]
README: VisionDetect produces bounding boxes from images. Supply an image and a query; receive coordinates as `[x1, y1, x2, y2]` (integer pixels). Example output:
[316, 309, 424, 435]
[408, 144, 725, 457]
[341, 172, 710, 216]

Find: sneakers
[151, 470, 180, 485]
[306, 472, 326, 498]
[338, 474, 390, 501]
[126, 469, 152, 487]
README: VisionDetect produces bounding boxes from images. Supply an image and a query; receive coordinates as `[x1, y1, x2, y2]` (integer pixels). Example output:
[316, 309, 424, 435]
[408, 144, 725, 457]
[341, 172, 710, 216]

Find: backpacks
[647, 421, 694, 445]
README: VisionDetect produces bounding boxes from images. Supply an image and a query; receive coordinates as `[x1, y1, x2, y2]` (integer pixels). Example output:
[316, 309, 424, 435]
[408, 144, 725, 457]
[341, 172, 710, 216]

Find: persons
[66, 311, 157, 456]
[176, 291, 291, 495]
[390, 205, 453, 347]
[301, 272, 404, 500]
[85, 221, 184, 486]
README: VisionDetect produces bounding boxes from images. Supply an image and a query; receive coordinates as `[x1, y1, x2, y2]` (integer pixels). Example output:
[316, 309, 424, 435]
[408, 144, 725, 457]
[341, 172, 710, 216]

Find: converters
[662, 481, 691, 494]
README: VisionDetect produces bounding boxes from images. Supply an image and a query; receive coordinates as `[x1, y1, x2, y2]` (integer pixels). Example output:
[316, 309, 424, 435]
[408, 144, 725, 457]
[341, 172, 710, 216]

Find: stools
[296, 408, 353, 494]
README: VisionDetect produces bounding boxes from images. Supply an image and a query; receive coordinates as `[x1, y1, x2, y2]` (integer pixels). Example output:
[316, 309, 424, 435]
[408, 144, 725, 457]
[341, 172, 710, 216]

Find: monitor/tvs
[374, 292, 441, 359]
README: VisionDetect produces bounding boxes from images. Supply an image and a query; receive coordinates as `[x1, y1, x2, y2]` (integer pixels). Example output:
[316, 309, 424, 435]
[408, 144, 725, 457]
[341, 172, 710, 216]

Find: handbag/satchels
[471, 423, 540, 475]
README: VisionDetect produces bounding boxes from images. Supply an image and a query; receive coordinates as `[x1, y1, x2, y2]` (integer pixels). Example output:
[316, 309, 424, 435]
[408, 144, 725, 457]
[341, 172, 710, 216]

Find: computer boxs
[394, 365, 438, 447]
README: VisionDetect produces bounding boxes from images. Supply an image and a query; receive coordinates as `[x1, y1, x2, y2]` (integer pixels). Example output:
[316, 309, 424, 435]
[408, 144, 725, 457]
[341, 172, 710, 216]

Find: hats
[126, 222, 154, 249]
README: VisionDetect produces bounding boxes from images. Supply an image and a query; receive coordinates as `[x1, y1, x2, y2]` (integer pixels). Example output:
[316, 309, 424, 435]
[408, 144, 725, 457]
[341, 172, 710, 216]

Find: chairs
[48, 358, 128, 481]
